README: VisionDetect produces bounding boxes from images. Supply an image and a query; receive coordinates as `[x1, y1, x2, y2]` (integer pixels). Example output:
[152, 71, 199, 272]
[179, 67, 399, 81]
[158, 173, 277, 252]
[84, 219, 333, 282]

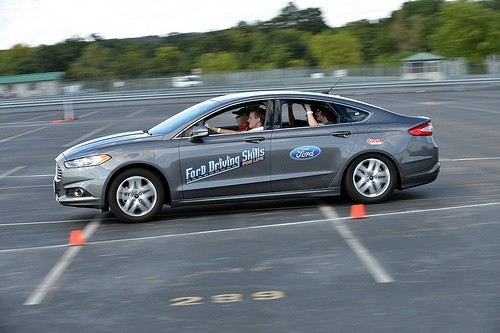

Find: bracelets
[288, 105, 292, 108]
[217, 128, 221, 132]
[307, 111, 314, 114]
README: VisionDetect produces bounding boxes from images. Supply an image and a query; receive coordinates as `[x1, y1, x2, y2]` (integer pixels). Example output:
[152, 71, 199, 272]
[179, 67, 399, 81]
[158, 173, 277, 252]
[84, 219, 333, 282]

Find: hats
[232, 108, 251, 116]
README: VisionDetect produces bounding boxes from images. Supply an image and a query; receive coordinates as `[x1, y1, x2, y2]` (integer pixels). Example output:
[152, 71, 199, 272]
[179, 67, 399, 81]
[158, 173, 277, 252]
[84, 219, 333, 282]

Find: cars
[51, 90, 442, 223]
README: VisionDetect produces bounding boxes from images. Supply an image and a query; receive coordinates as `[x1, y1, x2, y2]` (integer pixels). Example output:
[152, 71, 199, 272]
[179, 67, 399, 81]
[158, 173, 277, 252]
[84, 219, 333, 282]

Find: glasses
[317, 109, 321, 112]
[237, 115, 246, 118]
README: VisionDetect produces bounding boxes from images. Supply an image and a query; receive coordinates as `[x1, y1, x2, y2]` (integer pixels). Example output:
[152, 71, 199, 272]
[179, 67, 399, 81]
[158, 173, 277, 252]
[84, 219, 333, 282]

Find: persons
[231, 108, 252, 132]
[204, 108, 266, 133]
[286, 101, 322, 126]
[304, 104, 335, 126]
